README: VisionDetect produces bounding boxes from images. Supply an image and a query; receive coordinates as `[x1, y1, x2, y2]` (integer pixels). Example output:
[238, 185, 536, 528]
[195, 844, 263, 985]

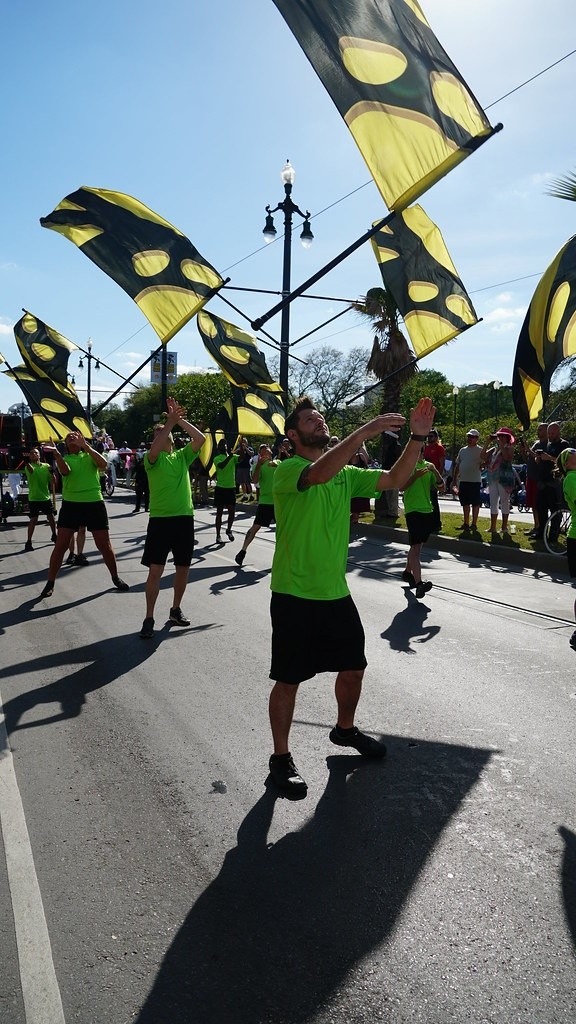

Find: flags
[197, 310, 284, 393]
[268, 1, 496, 207]
[511, 232, 576, 429]
[195, 408, 241, 468]
[14, 312, 79, 399]
[39, 182, 224, 346]
[229, 387, 287, 437]
[368, 205, 479, 360]
[0, 352, 92, 443]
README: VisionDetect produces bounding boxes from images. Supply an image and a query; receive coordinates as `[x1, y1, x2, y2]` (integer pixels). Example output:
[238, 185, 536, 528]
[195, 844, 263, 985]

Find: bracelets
[411, 434, 428, 442]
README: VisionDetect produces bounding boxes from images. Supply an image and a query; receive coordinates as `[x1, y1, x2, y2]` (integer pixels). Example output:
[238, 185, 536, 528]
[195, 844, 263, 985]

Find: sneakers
[139, 618, 154, 637]
[329, 725, 386, 759]
[169, 607, 190, 625]
[270, 753, 308, 791]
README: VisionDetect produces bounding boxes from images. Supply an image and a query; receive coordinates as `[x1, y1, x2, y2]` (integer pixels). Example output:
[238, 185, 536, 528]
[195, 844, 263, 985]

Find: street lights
[262, 160, 314, 415]
[78, 336, 100, 422]
[453, 385, 459, 501]
[493, 380, 501, 421]
[341, 401, 346, 441]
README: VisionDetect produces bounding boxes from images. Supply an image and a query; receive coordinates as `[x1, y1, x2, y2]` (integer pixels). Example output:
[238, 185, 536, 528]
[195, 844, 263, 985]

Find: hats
[496, 428, 515, 444]
[466, 429, 480, 437]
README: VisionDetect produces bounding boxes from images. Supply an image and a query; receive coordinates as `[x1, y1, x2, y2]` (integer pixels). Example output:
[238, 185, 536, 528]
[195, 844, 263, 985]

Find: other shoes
[469, 525, 477, 530]
[456, 524, 469, 530]
[25, 495, 254, 596]
[416, 581, 432, 598]
[524, 528, 535, 535]
[402, 571, 415, 587]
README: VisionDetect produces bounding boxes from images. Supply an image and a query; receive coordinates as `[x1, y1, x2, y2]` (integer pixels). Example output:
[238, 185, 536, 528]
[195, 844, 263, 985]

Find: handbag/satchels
[500, 463, 515, 486]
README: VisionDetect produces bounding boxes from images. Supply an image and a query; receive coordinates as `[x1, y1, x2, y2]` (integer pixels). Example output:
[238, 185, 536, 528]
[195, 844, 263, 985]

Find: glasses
[565, 448, 576, 462]
[331, 441, 337, 443]
[428, 435, 434, 437]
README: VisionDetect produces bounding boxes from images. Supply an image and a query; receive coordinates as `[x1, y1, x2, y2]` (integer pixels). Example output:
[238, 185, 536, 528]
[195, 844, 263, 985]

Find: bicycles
[542, 507, 573, 556]
[104, 477, 114, 496]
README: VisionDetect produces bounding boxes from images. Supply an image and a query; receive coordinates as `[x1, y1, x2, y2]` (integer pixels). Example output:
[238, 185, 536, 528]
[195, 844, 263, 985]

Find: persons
[40, 432, 129, 597]
[23, 422, 576, 650]
[140, 396, 207, 634]
[7, 473, 22, 499]
[269, 397, 436, 795]
